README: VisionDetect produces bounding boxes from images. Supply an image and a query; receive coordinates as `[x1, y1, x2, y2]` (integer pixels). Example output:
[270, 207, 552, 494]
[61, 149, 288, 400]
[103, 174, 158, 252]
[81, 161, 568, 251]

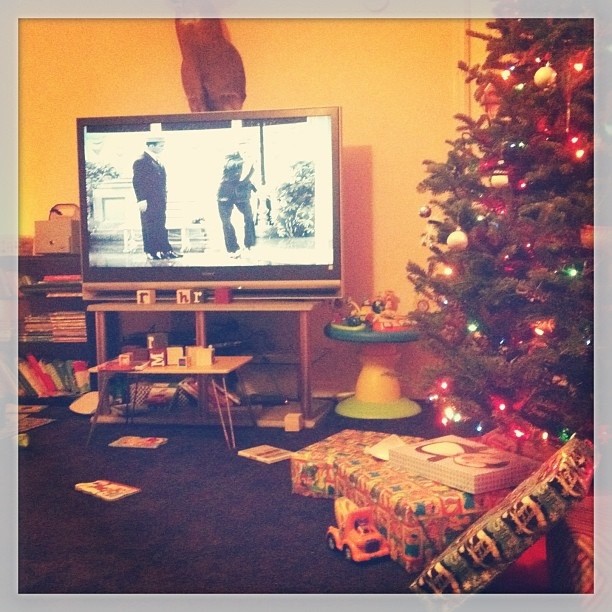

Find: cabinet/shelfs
[87, 300, 336, 431]
[17, 250, 94, 406]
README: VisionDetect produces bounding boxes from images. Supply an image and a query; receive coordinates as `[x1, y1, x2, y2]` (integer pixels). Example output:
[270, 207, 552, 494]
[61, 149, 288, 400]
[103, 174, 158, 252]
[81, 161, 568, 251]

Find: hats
[146, 132, 166, 143]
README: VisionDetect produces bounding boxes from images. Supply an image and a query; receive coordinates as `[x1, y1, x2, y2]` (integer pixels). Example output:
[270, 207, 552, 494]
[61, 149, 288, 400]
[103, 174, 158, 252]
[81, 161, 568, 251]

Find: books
[144, 382, 178, 406]
[19, 310, 86, 342]
[76, 479, 142, 502]
[108, 435, 168, 449]
[43, 274, 80, 282]
[178, 377, 240, 409]
[19, 354, 89, 398]
[238, 443, 293, 463]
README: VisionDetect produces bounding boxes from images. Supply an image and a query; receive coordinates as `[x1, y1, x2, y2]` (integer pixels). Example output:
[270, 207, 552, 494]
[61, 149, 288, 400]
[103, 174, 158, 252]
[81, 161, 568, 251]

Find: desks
[87, 352, 253, 447]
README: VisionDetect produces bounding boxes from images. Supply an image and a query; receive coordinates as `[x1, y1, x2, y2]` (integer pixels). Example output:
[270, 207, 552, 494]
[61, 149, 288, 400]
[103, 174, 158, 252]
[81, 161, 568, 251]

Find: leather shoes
[146, 252, 160, 259]
[161, 250, 183, 259]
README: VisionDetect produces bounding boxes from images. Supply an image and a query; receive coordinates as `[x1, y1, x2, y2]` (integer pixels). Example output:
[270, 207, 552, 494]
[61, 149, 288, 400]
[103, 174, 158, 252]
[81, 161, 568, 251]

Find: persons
[132, 133, 185, 261]
[217, 141, 260, 259]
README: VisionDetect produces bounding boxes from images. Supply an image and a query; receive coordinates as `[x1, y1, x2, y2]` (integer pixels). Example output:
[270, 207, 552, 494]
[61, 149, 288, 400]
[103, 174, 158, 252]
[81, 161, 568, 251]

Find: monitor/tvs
[75, 106, 343, 304]
